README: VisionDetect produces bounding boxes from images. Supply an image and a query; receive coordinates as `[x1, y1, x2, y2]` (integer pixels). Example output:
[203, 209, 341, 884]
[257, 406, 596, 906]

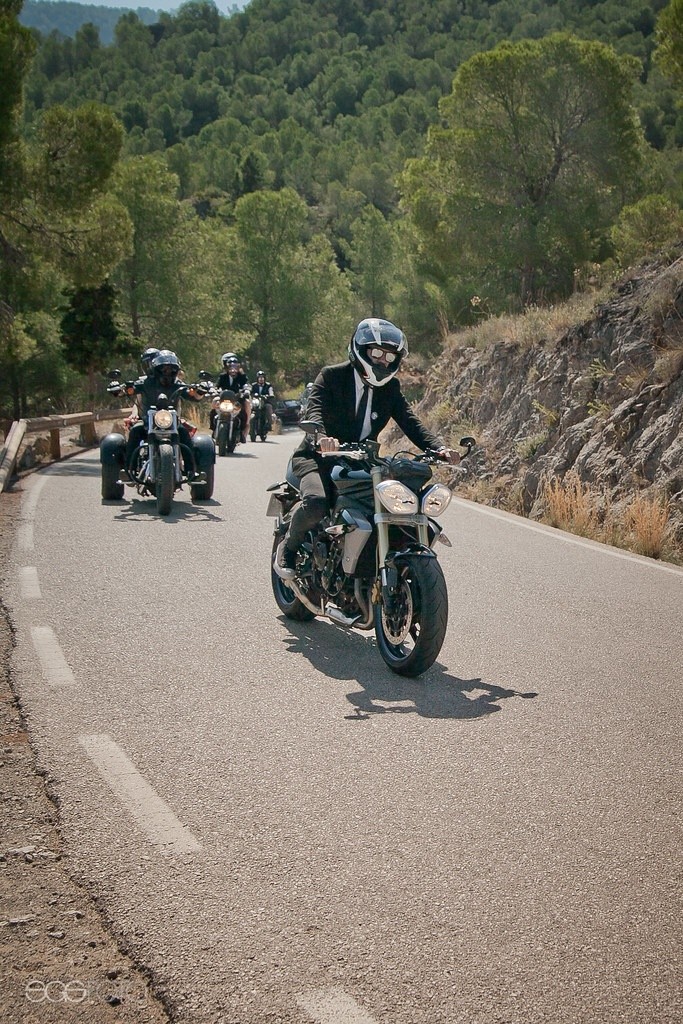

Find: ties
[354, 385, 369, 441]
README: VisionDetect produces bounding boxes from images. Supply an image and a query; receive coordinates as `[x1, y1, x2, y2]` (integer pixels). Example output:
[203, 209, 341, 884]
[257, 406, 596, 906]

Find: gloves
[196, 381, 213, 396]
[269, 396, 274, 401]
[244, 393, 249, 399]
[107, 381, 120, 397]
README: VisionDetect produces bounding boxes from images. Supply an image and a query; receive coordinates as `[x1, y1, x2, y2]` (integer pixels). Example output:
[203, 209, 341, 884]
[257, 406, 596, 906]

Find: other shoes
[211, 431, 216, 439]
[188, 472, 207, 482]
[119, 469, 135, 487]
[264, 423, 271, 431]
[240, 434, 246, 443]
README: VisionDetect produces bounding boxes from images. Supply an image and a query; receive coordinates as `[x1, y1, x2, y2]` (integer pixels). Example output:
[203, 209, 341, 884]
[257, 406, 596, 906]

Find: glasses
[365, 345, 398, 363]
[154, 365, 178, 376]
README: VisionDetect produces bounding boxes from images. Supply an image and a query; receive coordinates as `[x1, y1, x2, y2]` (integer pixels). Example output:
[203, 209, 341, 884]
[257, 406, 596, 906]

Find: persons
[273, 318, 461, 580]
[120, 346, 207, 488]
[209, 352, 251, 444]
[249, 370, 275, 436]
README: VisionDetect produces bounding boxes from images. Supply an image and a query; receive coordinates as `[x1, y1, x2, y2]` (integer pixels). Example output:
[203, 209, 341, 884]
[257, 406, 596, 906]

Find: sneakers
[273, 540, 296, 580]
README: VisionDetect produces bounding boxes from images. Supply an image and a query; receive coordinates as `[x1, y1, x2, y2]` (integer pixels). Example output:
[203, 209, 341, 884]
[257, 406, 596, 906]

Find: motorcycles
[100, 369, 217, 516]
[263, 420, 476, 677]
[246, 392, 272, 443]
[197, 369, 251, 456]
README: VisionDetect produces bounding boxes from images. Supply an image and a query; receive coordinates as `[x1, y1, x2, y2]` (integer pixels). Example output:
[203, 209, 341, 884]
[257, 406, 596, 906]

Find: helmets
[347, 317, 409, 388]
[221, 353, 238, 369]
[256, 371, 266, 380]
[140, 348, 159, 374]
[149, 350, 181, 371]
[226, 357, 240, 366]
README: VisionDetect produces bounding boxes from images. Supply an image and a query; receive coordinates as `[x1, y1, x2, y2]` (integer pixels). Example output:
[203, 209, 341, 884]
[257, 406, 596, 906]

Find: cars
[276, 399, 305, 427]
